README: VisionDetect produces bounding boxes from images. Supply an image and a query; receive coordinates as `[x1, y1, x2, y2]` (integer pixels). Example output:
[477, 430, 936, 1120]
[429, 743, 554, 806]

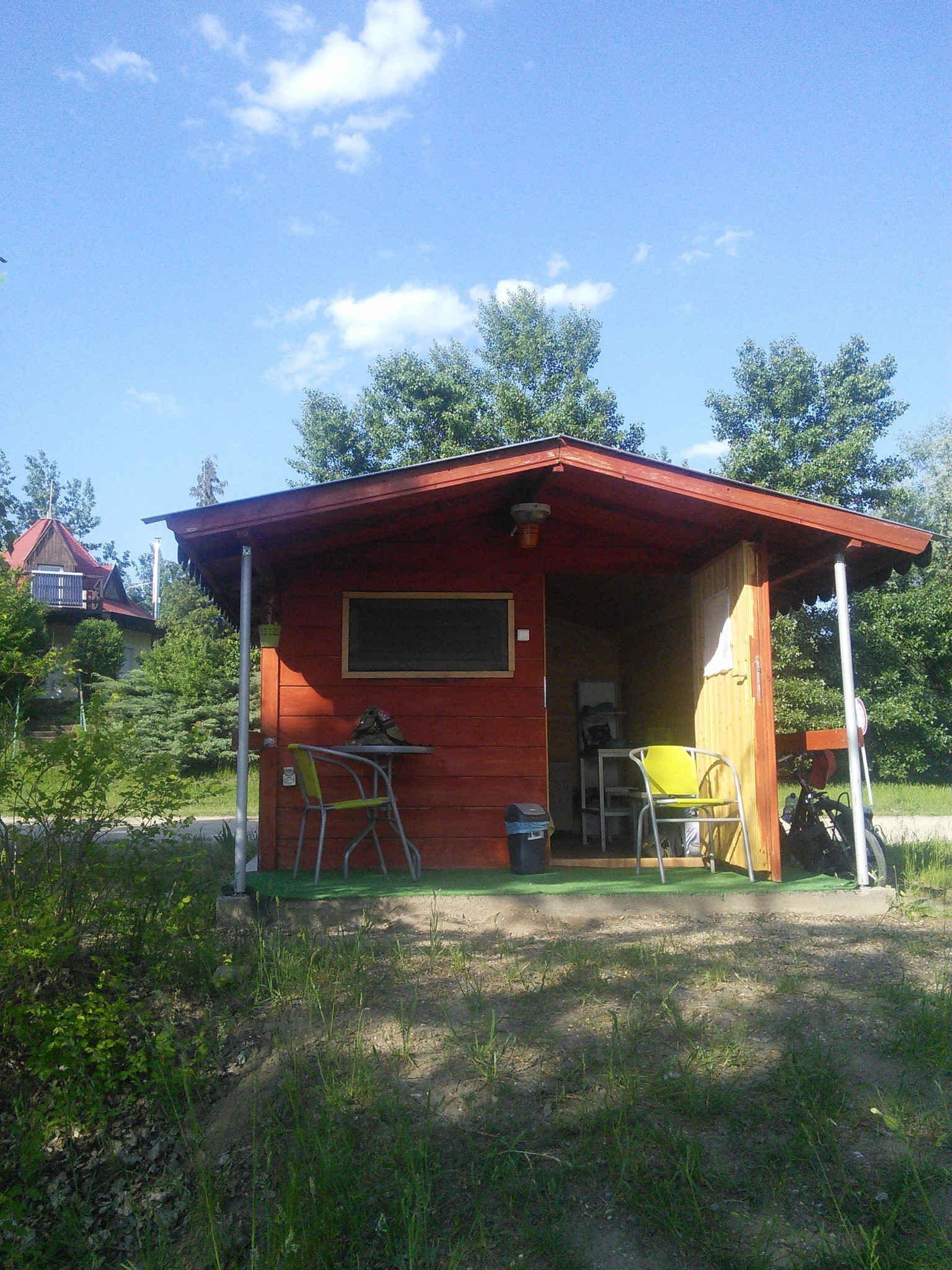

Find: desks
[332, 744, 435, 880]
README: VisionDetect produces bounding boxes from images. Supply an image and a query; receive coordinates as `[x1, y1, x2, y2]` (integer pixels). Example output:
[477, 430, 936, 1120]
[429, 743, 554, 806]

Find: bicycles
[789, 773, 888, 887]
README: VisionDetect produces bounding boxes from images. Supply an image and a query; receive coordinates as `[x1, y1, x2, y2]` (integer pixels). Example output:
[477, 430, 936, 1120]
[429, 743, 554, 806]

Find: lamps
[509, 494, 550, 549]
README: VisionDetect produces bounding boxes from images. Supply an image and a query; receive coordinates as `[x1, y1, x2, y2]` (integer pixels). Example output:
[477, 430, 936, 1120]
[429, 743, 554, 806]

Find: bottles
[784, 793, 798, 821]
[377, 707, 391, 722]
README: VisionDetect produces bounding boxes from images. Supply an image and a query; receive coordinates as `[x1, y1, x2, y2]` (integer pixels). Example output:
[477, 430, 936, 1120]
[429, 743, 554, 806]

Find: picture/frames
[341, 592, 516, 679]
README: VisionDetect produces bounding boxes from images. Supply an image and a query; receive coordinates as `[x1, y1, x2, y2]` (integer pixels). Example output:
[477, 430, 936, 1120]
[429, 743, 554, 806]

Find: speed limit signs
[855, 697, 867, 736]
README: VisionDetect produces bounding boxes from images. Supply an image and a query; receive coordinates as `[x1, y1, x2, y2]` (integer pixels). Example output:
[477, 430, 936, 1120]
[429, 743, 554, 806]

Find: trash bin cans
[504, 803, 551, 875]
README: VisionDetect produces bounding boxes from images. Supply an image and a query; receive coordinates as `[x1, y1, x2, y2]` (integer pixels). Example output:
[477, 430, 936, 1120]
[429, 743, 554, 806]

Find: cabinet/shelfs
[576, 676, 649, 854]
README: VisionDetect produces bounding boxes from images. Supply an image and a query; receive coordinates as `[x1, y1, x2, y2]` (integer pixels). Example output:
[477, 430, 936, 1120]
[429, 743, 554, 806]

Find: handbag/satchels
[349, 704, 406, 746]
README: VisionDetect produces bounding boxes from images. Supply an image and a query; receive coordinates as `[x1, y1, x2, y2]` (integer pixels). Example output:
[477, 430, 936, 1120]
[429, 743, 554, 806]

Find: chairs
[629, 745, 755, 884]
[289, 744, 424, 885]
